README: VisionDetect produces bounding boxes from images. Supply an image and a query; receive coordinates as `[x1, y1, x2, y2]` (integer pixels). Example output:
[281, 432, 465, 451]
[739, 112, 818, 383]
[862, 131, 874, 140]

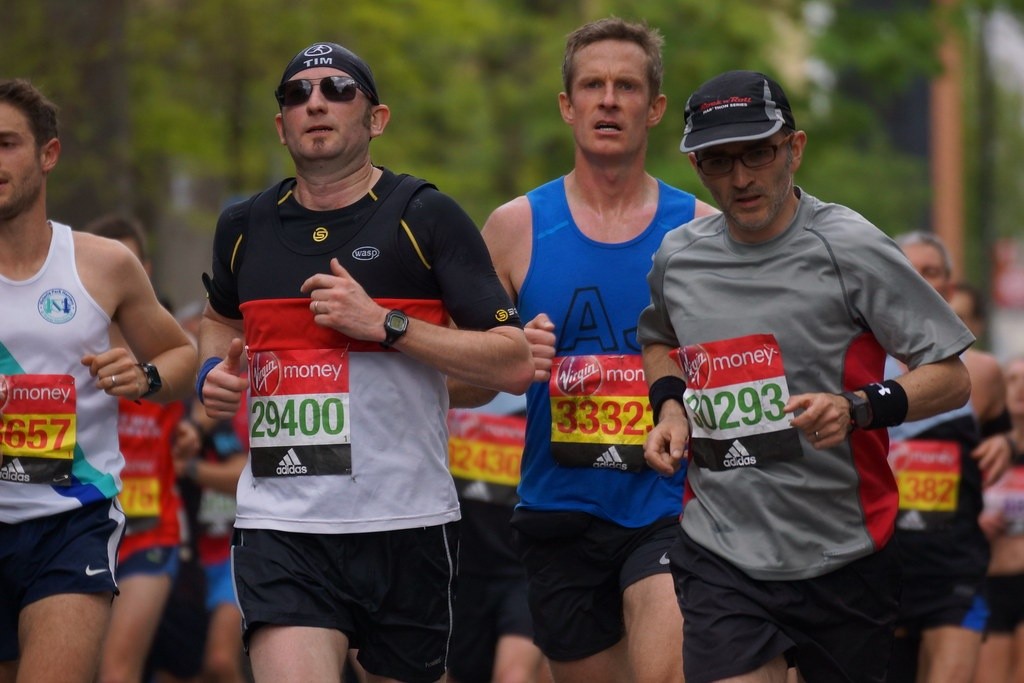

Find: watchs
[840, 392, 869, 430]
[382, 309, 408, 346]
[133, 363, 162, 405]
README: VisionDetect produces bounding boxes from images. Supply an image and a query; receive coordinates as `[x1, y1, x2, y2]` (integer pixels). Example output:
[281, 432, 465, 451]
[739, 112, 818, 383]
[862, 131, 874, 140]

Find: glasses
[276, 73, 377, 105]
[694, 131, 795, 177]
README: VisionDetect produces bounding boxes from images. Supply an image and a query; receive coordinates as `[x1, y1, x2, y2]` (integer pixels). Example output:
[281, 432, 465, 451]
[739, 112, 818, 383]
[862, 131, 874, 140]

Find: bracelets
[864, 380, 908, 429]
[197, 356, 225, 406]
[648, 376, 686, 427]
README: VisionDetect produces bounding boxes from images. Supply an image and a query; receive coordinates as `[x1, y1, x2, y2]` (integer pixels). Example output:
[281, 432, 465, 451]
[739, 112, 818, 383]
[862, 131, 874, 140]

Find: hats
[678, 69, 796, 154]
[281, 42, 379, 105]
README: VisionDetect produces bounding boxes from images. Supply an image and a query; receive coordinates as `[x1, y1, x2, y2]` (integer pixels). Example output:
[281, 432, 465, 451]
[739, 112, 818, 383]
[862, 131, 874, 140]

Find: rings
[112, 376, 117, 385]
[815, 431, 820, 441]
[315, 301, 320, 313]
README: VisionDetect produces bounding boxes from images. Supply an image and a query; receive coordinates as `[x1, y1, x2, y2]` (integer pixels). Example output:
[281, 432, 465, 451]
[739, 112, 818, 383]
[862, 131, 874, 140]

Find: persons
[637, 70, 1024, 683]
[195, 41, 534, 683]
[438, 16, 724, 683]
[0, 79, 253, 683]
